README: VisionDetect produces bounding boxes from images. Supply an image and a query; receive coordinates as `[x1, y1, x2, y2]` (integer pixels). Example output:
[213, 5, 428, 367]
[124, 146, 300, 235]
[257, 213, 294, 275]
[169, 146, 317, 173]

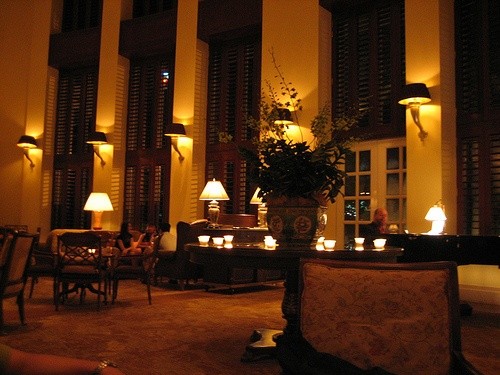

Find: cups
[373, 239, 387, 248]
[323, 240, 336, 249]
[212, 238, 224, 244]
[197, 236, 211, 244]
[354, 238, 365, 245]
[224, 235, 234, 242]
[263, 236, 277, 249]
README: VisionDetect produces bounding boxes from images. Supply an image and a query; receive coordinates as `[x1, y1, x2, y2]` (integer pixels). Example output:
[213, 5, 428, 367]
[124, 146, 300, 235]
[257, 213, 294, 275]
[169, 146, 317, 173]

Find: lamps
[198, 178, 230, 229]
[398, 83, 432, 141]
[82, 192, 115, 230]
[424, 205, 447, 234]
[271, 108, 293, 129]
[249, 187, 268, 227]
[17, 135, 38, 169]
[165, 124, 185, 162]
[86, 131, 108, 168]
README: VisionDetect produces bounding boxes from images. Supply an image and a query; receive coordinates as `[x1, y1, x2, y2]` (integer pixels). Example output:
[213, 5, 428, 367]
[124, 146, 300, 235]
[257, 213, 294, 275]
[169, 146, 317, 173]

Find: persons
[113, 221, 137, 256]
[364, 207, 389, 234]
[0, 344, 128, 375]
[137, 221, 177, 276]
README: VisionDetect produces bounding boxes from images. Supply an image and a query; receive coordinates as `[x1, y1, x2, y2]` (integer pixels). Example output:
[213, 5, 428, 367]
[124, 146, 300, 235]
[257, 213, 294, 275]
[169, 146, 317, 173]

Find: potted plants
[217, 47, 364, 244]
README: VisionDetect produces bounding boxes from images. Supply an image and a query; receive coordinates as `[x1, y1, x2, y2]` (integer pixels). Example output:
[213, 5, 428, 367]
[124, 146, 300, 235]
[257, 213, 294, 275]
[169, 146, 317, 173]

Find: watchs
[95, 358, 118, 375]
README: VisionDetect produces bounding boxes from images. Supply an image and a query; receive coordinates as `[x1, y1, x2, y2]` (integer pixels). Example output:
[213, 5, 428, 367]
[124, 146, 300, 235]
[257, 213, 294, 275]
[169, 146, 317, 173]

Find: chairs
[280, 258, 482, 375]
[0, 218, 208, 336]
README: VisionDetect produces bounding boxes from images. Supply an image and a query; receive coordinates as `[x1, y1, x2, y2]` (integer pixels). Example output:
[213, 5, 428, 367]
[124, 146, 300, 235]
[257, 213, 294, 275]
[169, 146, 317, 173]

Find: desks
[310, 245, 404, 264]
[184, 243, 280, 290]
